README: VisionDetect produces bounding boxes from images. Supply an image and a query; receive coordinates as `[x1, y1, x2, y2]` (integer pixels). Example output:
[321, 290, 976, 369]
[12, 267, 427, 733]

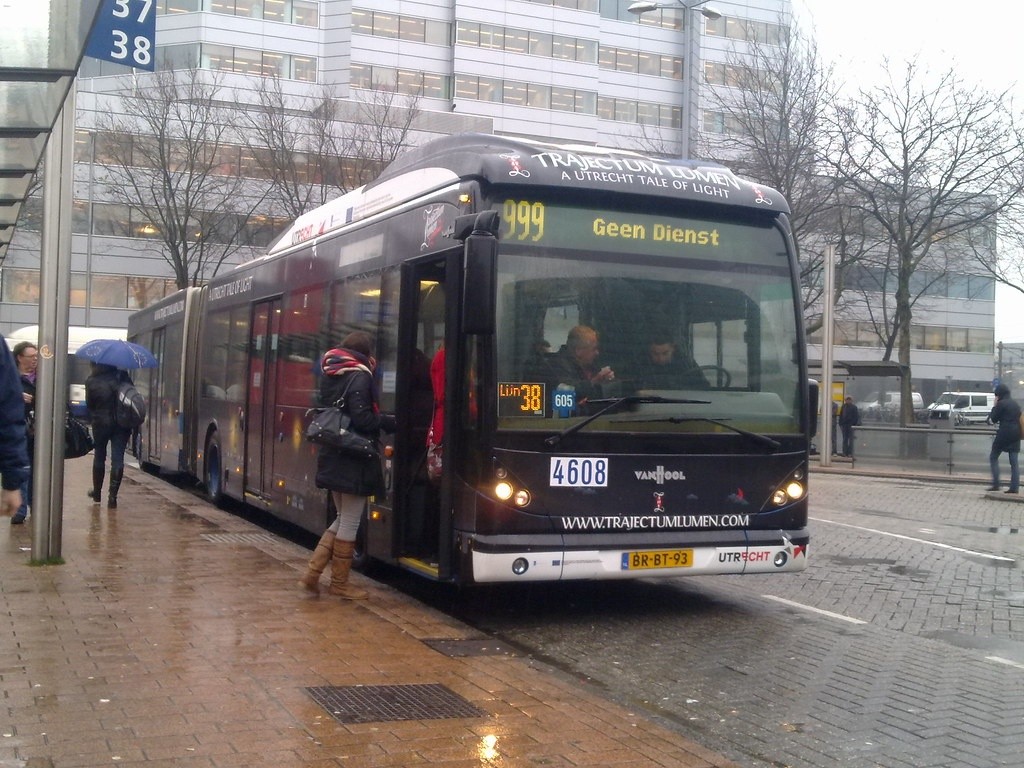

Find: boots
[297, 529, 336, 594]
[329, 539, 368, 600]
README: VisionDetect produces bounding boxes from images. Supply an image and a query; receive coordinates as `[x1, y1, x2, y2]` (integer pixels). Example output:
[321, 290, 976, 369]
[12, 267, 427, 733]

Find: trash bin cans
[927, 409, 955, 461]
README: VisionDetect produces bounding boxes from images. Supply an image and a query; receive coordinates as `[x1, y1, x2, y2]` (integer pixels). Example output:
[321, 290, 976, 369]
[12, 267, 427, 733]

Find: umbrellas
[75, 339, 158, 369]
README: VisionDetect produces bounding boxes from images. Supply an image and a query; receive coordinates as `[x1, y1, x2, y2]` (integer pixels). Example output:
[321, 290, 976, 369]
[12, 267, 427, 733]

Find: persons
[299, 333, 380, 600]
[0, 333, 28, 516]
[839, 397, 858, 457]
[542, 326, 615, 415]
[819, 399, 838, 456]
[986, 384, 1022, 493]
[427, 344, 479, 566]
[86, 361, 135, 508]
[622, 333, 711, 394]
[10, 342, 37, 524]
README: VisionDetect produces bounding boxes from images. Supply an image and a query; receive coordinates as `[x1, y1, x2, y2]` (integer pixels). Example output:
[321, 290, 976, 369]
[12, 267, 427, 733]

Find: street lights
[626, 1, 722, 160]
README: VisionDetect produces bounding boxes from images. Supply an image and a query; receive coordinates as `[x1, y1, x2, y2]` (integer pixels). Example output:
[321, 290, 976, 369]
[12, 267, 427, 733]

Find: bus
[126, 131, 818, 591]
[5, 325, 128, 418]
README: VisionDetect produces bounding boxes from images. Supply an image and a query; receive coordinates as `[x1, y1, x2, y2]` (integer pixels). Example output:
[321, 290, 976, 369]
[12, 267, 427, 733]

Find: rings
[609, 378, 612, 381]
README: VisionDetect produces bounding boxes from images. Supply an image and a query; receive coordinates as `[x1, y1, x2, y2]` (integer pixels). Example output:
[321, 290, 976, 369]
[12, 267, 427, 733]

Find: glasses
[22, 354, 37, 358]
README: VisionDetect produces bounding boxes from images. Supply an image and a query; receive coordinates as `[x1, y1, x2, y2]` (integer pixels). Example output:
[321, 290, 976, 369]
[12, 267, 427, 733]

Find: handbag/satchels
[1019, 415, 1024, 439]
[62, 401, 93, 461]
[426, 444, 442, 479]
[856, 417, 862, 425]
[306, 371, 376, 457]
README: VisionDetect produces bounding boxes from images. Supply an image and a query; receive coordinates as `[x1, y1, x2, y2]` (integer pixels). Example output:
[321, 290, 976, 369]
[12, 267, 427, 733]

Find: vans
[925, 392, 997, 427]
[855, 391, 926, 418]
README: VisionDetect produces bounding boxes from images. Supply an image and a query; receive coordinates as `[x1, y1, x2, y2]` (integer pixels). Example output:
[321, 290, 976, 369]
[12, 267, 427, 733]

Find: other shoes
[1005, 489, 1018, 493]
[89, 490, 101, 502]
[987, 487, 999, 491]
[430, 556, 439, 567]
[11, 513, 25, 526]
[108, 496, 117, 508]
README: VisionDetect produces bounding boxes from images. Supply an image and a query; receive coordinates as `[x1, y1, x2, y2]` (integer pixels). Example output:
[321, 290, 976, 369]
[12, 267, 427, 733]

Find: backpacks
[113, 370, 147, 430]
